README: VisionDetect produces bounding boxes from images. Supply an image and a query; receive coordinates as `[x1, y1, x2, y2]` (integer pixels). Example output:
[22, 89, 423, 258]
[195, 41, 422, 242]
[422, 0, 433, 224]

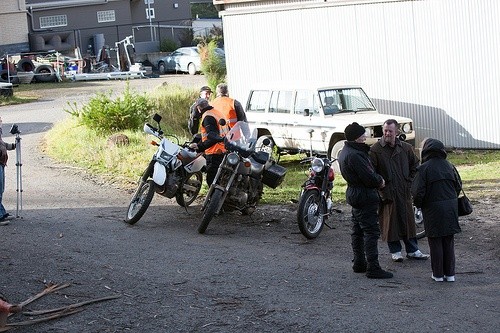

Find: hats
[344, 122, 366, 141]
[200, 86, 213, 93]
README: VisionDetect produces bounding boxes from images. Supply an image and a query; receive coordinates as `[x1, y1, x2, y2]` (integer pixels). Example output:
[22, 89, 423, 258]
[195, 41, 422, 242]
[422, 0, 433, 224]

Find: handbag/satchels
[458, 195, 473, 217]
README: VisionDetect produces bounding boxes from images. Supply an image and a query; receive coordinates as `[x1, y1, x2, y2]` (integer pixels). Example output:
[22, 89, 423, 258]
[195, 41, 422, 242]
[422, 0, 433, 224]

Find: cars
[155, 46, 225, 75]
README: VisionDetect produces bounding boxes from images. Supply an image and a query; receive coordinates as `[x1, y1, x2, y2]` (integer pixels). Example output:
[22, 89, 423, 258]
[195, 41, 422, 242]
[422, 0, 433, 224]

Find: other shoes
[0, 217, 10, 225]
[392, 251, 403, 261]
[431, 272, 443, 282]
[352, 259, 367, 272]
[408, 250, 430, 259]
[4, 213, 14, 220]
[444, 275, 455, 281]
[366, 265, 393, 278]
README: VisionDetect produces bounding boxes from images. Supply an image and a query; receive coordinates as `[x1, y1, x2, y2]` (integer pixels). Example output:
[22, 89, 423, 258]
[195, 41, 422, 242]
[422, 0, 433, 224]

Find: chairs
[324, 97, 339, 115]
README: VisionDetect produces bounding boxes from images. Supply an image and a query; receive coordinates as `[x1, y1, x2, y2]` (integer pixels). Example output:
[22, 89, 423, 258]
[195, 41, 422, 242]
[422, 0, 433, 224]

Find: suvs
[244, 84, 416, 162]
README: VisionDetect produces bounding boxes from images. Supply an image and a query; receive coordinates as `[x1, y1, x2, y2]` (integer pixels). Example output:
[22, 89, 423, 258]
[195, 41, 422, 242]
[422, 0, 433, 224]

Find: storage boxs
[262, 164, 287, 189]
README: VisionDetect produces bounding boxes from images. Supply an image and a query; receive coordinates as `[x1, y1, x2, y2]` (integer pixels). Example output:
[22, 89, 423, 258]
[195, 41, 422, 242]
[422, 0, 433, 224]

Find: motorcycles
[291, 145, 339, 240]
[196, 117, 286, 234]
[125, 112, 205, 232]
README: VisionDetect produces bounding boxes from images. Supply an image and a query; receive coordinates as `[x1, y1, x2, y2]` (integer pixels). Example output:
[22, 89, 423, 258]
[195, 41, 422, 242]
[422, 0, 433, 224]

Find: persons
[410, 138, 462, 283]
[188, 83, 248, 216]
[372, 119, 429, 262]
[0, 117, 16, 225]
[339, 123, 393, 279]
